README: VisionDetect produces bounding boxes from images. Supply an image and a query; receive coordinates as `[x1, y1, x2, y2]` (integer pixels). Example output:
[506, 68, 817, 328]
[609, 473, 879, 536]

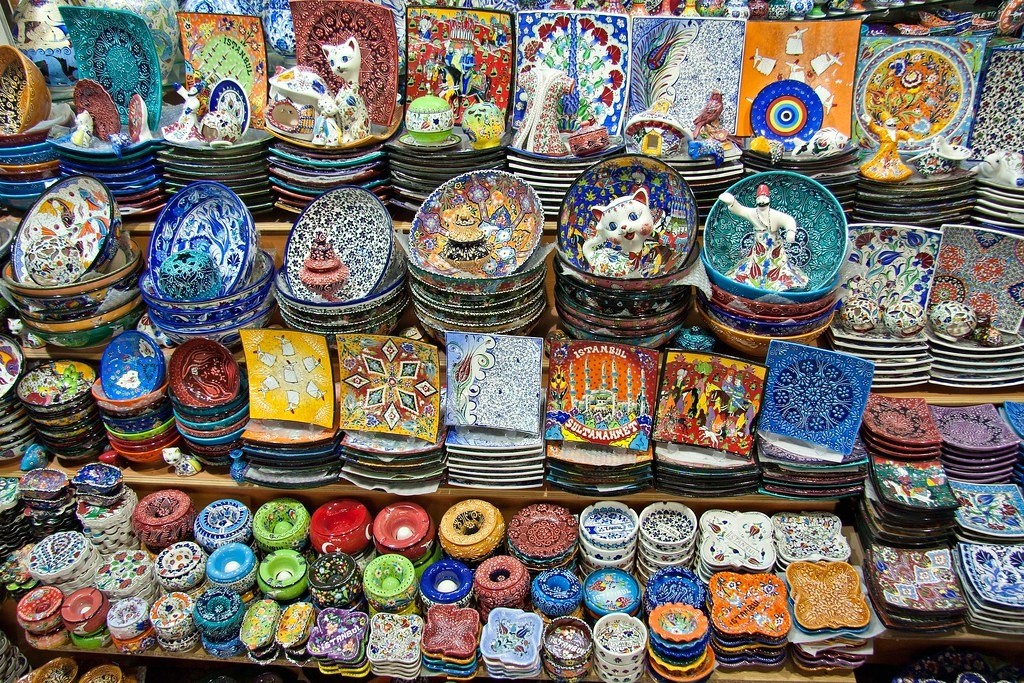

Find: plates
[0, 0, 1024, 683]
